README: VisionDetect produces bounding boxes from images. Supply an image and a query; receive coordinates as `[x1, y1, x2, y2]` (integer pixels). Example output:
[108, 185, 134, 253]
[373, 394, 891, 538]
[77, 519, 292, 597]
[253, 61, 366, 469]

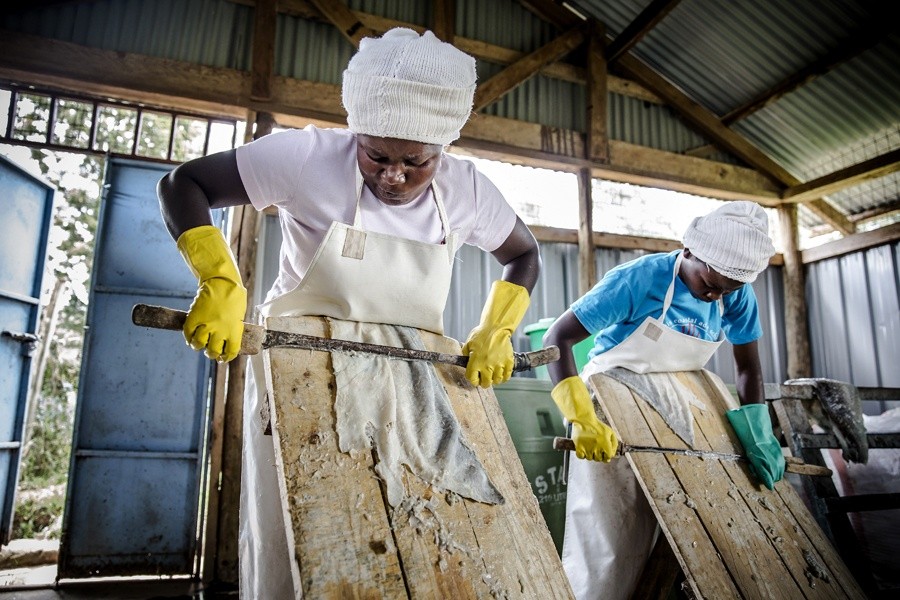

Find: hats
[340, 27, 477, 145]
[681, 201, 776, 283]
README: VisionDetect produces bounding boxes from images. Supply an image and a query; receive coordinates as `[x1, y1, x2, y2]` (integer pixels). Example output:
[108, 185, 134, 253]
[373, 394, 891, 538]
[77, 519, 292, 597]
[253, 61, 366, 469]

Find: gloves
[725, 403, 786, 490]
[550, 375, 618, 463]
[461, 280, 531, 389]
[176, 225, 247, 363]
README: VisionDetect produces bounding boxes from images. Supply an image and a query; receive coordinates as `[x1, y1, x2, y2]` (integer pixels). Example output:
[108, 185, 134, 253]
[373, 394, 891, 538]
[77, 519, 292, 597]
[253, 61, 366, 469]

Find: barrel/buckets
[523, 316, 599, 381]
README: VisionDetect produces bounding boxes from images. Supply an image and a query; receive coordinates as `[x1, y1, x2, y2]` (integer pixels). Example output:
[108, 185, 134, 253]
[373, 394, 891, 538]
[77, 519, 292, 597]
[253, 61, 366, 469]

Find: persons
[156, 27, 542, 599]
[542, 201, 777, 600]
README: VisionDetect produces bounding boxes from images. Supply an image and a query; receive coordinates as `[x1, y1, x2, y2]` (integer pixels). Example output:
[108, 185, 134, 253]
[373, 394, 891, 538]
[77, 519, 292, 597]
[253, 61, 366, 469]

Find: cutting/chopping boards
[587, 366, 867, 600]
[260, 316, 578, 600]
